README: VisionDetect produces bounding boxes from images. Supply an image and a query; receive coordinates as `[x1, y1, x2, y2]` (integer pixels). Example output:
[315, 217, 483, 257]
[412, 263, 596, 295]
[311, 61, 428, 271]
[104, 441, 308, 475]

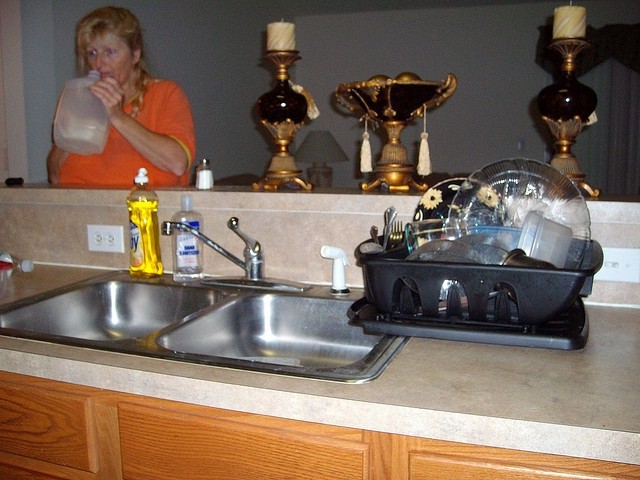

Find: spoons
[384, 206, 398, 249]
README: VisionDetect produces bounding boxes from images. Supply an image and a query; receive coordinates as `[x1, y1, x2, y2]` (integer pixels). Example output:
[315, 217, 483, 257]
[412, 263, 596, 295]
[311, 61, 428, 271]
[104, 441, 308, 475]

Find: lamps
[294, 130, 350, 186]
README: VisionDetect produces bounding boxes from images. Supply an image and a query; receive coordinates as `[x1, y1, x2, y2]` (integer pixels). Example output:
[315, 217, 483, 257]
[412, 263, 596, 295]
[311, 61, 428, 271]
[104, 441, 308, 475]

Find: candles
[266, 17, 297, 51]
[552, 0, 588, 39]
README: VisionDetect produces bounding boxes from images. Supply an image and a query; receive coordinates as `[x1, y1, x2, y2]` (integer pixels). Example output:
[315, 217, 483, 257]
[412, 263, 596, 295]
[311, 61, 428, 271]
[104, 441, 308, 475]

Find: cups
[405, 220, 452, 255]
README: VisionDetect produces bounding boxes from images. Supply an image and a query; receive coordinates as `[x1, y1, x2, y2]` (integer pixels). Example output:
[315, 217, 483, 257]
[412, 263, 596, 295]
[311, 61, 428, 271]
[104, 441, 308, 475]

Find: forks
[388, 221, 403, 249]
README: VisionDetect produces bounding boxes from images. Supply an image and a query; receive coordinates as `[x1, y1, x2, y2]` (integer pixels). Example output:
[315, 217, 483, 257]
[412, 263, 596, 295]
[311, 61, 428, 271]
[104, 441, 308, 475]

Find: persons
[46, 6, 198, 189]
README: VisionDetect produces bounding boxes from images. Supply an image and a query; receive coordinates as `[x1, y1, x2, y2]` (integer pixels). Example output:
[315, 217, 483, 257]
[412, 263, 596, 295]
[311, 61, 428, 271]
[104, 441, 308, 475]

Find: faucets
[320, 245, 349, 292]
[161, 217, 263, 285]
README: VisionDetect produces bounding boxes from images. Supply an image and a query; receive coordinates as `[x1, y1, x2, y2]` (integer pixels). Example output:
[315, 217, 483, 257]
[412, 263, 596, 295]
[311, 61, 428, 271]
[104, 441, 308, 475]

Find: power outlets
[87, 224, 126, 254]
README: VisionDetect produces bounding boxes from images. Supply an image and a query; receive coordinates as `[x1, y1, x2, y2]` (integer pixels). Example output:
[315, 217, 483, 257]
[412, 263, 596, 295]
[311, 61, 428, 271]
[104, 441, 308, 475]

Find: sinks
[156, 293, 388, 370]
[1, 280, 226, 342]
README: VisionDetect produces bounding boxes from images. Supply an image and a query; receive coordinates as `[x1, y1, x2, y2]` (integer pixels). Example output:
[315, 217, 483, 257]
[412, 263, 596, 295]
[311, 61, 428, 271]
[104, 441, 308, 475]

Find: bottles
[196, 158, 214, 190]
[0, 251, 34, 279]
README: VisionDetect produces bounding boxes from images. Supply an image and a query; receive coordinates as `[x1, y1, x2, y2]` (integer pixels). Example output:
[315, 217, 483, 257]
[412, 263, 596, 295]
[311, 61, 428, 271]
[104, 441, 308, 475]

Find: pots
[374, 248, 560, 321]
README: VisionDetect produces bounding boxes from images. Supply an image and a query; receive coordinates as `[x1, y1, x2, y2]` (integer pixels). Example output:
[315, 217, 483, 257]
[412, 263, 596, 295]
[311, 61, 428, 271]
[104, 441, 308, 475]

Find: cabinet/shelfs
[0, 370, 640, 480]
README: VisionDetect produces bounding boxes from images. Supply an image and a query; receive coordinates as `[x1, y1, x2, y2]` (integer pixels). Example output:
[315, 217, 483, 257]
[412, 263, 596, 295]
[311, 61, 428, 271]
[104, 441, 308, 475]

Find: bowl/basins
[453, 233, 509, 285]
[402, 240, 488, 302]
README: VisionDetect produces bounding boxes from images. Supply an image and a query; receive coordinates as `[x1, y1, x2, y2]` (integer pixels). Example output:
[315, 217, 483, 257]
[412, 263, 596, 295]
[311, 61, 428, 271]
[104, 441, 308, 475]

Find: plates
[413, 178, 510, 247]
[448, 158, 591, 269]
